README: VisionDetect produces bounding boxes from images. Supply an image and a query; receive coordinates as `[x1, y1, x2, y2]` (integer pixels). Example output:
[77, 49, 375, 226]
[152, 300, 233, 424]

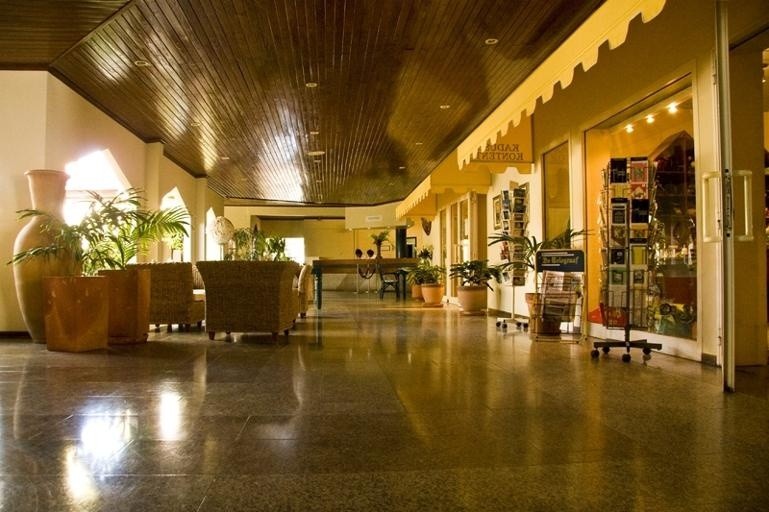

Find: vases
[13, 170, 84, 345]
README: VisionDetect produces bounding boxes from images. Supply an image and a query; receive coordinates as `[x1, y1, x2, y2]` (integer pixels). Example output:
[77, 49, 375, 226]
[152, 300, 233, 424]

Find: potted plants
[6, 187, 194, 354]
[370, 229, 394, 260]
[402, 220, 594, 335]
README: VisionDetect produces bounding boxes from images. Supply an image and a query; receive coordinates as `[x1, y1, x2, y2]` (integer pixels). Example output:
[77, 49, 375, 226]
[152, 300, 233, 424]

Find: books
[499, 188, 528, 287]
[598, 155, 658, 330]
[534, 270, 581, 322]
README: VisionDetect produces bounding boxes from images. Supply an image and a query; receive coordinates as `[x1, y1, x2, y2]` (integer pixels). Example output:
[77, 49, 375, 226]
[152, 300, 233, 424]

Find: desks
[312, 257, 422, 310]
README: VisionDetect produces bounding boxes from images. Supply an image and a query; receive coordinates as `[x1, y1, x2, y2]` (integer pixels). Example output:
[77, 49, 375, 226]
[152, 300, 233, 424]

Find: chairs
[125, 261, 312, 348]
